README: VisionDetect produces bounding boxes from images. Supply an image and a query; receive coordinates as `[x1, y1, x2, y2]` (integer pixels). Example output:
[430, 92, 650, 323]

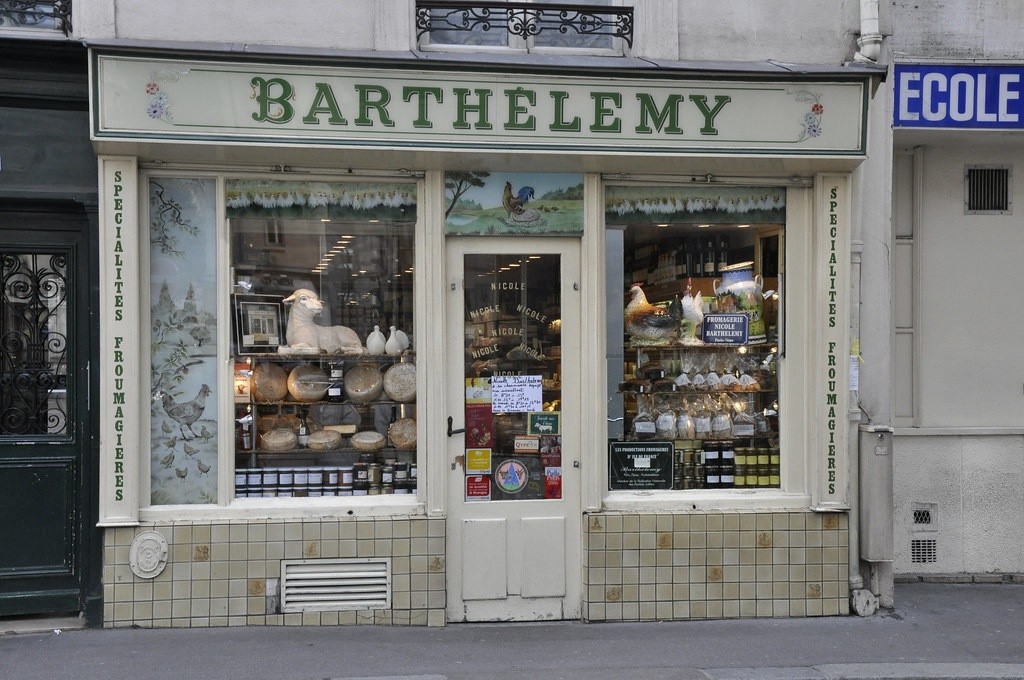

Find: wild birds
[365, 325, 409, 356]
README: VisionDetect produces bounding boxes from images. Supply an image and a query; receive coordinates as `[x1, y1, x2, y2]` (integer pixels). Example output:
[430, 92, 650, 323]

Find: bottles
[717, 237, 728, 276]
[297, 412, 311, 449]
[704, 240, 718, 278]
[692, 243, 704, 278]
[239, 408, 253, 451]
[632, 241, 690, 283]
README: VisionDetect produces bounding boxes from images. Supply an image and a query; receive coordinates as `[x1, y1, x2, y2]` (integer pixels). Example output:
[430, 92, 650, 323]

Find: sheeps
[278, 288, 367, 355]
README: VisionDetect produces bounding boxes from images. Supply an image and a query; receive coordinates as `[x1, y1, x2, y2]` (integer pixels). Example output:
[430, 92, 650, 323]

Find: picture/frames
[233, 294, 286, 355]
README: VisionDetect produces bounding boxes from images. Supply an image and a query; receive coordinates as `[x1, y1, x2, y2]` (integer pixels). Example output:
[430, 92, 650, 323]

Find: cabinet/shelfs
[465, 320, 561, 500]
[623, 333, 782, 490]
[231, 354, 416, 499]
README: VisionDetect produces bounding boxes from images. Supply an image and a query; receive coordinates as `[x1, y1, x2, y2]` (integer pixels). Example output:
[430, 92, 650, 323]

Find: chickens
[682, 277, 703, 338]
[624, 282, 682, 347]
